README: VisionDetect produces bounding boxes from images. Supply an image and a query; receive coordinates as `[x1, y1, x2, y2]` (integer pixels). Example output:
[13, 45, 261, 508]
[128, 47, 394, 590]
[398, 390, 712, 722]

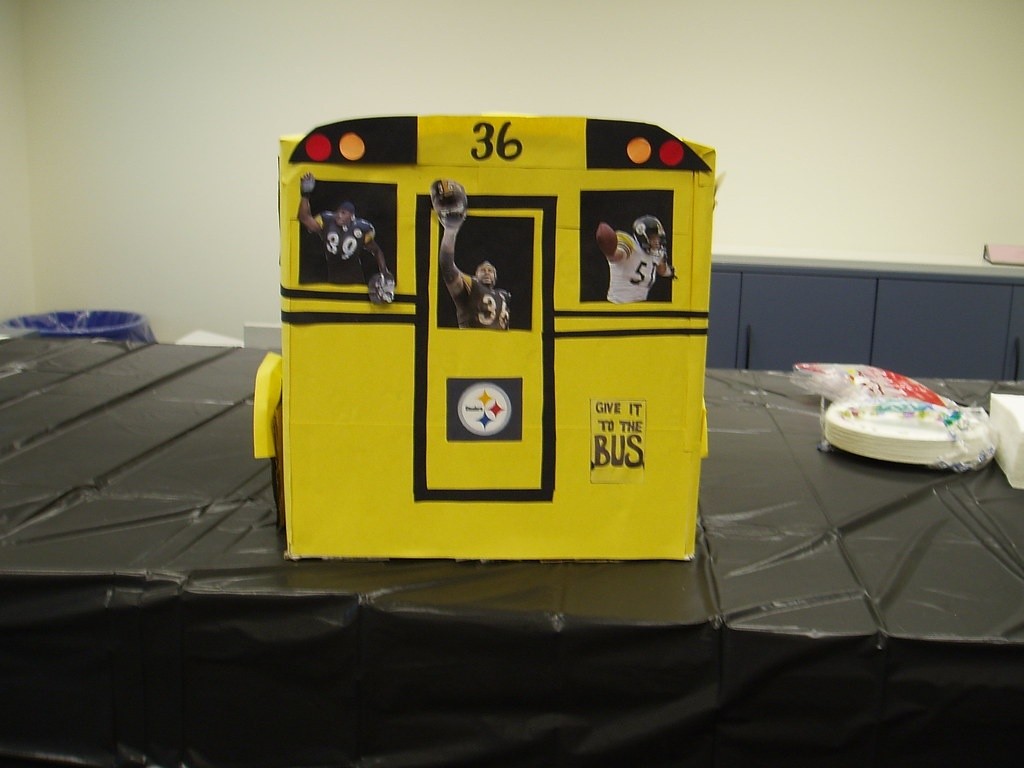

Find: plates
[825, 399, 990, 464]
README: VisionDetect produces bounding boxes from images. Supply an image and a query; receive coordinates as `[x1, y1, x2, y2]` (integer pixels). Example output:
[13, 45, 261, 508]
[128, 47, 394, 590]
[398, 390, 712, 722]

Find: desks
[0, 334, 1024, 768]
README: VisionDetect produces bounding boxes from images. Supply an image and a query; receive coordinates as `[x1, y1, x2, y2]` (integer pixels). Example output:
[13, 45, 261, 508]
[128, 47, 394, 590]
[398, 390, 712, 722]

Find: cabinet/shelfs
[703, 258, 1024, 382]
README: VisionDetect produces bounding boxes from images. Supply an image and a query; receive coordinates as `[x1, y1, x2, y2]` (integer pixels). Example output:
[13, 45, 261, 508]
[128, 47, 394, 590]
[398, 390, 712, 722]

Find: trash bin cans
[1, 309, 157, 344]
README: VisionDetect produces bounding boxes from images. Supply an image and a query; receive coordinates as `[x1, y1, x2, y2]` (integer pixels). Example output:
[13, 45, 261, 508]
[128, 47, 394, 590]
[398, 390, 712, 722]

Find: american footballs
[596, 222, 617, 256]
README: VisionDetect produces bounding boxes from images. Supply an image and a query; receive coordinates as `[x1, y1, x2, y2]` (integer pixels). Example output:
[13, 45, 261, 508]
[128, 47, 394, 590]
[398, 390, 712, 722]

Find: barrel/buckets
[2, 310, 156, 343]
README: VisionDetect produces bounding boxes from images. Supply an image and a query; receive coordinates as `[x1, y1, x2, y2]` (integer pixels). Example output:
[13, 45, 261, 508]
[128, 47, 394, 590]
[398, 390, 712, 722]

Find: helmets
[632, 216, 661, 249]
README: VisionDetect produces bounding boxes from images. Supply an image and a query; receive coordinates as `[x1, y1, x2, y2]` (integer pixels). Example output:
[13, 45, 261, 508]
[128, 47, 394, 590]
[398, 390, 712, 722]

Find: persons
[297, 173, 393, 307]
[598, 217, 673, 302]
[431, 178, 511, 332]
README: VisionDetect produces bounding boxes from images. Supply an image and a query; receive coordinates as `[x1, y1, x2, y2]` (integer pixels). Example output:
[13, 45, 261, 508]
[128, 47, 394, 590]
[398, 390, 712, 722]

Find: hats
[339, 202, 355, 214]
